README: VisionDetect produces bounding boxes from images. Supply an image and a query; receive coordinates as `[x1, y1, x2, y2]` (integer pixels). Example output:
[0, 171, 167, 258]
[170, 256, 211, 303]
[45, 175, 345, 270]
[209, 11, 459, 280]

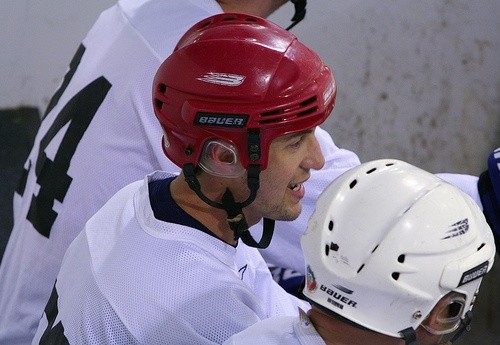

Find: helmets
[488, 146, 500, 202]
[152, 12, 335, 169]
[302, 159, 496, 339]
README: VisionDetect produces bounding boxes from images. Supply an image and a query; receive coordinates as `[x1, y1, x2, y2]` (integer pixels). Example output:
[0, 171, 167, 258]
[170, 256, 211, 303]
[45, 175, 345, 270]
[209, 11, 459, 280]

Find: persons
[222, 159, 497, 345]
[0, 0, 500, 345]
[30, 14, 336, 345]
